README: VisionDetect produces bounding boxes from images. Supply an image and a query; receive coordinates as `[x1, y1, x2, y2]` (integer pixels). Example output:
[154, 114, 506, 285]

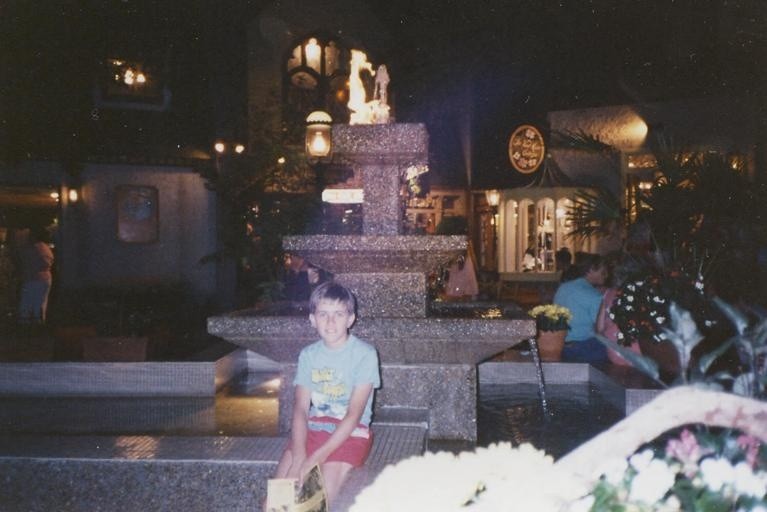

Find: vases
[535, 330, 568, 362]
[654, 303, 707, 388]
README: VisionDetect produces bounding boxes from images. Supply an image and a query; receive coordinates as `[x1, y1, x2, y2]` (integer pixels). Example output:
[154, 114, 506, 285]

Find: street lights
[487, 185, 502, 280]
[304, 109, 336, 233]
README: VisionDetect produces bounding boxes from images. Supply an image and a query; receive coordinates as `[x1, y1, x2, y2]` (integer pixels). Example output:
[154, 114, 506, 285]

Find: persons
[596, 277, 644, 368]
[17, 226, 54, 326]
[262, 279, 382, 512]
[551, 256, 612, 361]
[554, 248, 573, 270]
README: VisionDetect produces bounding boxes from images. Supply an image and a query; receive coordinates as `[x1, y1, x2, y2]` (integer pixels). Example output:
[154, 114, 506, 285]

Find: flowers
[345, 438, 598, 512]
[602, 404, 765, 512]
[529, 301, 573, 331]
[609, 265, 718, 348]
